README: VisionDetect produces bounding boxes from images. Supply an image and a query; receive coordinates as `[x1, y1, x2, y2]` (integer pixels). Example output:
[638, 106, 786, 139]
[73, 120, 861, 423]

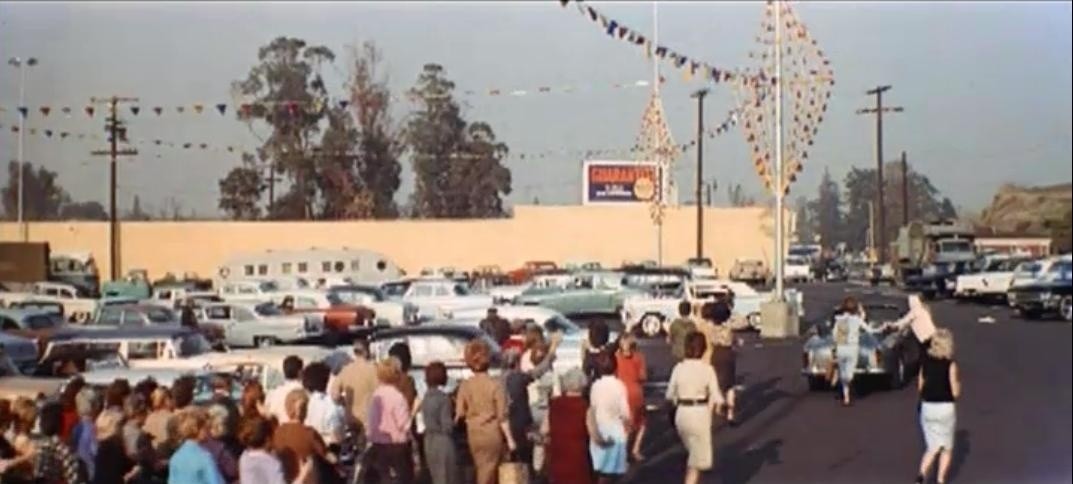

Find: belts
[677, 399, 708, 405]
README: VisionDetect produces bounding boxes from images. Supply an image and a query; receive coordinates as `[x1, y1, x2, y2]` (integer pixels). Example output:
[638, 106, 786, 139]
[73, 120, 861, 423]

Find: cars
[1008, 258, 1073, 323]
[801, 301, 922, 391]
[688, 240, 852, 287]
[621, 278, 805, 339]
[891, 216, 1035, 308]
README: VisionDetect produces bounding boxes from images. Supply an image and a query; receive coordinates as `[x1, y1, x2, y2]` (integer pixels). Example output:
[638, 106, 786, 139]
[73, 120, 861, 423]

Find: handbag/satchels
[498, 462, 530, 483]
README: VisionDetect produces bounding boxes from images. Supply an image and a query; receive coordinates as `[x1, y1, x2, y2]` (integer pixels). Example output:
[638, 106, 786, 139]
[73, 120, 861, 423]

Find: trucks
[0, 240, 99, 299]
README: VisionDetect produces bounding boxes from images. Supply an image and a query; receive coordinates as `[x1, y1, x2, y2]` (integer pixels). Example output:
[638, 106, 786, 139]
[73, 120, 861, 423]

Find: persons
[663, 332, 726, 484]
[824, 292, 937, 405]
[669, 293, 739, 421]
[1, 276, 652, 482]
[754, 241, 936, 287]
[915, 328, 960, 484]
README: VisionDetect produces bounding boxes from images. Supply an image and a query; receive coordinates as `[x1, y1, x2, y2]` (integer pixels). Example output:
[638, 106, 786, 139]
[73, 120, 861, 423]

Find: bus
[213, 245, 409, 294]
